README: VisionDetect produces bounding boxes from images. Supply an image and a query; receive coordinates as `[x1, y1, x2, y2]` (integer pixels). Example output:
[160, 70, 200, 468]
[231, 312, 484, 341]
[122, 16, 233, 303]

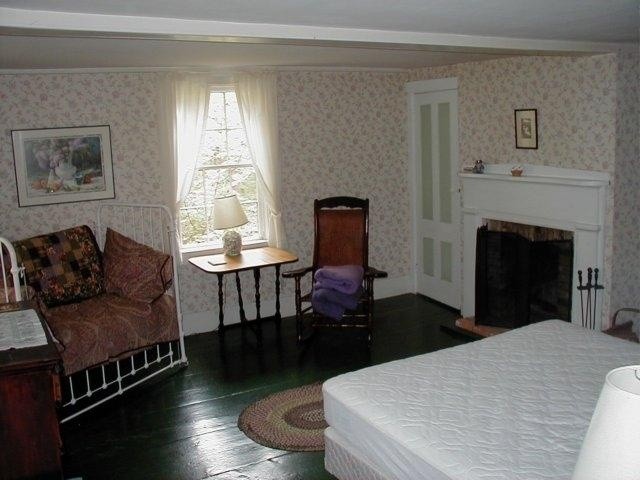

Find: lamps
[214, 195, 249, 257]
[571, 365, 640, 480]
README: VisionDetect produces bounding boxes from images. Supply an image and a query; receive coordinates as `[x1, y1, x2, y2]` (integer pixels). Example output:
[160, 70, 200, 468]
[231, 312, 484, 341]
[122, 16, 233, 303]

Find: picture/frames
[514, 109, 538, 150]
[11, 125, 116, 208]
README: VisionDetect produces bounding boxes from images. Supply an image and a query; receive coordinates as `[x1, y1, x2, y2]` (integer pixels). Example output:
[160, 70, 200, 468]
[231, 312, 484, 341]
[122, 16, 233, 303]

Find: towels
[309, 262, 366, 322]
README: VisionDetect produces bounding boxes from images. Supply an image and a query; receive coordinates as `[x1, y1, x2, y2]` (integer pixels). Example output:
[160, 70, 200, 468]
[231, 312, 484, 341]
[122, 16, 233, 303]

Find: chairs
[282, 196, 387, 342]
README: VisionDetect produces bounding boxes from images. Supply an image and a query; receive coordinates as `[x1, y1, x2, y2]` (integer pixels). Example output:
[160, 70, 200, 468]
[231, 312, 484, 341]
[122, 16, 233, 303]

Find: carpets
[238, 381, 328, 451]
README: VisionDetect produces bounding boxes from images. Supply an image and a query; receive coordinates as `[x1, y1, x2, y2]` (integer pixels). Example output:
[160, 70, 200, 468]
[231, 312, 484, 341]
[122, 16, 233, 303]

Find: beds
[323, 319, 640, 480]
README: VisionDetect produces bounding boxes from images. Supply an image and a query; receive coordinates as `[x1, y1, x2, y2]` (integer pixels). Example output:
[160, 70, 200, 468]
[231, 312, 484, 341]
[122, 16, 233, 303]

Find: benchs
[0, 204, 188, 424]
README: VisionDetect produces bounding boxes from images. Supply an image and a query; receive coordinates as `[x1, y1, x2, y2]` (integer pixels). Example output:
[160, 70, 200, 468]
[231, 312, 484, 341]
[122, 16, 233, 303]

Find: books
[208, 259, 228, 266]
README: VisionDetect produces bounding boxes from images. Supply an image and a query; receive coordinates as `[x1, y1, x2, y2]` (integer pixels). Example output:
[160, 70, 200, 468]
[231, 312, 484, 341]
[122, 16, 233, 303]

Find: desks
[0, 301, 65, 480]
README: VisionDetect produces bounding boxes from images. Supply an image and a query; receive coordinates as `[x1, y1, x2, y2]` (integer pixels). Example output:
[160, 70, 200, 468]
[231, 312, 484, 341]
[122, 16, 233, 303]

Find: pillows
[102, 228, 174, 302]
[0, 286, 65, 350]
[0, 225, 105, 307]
